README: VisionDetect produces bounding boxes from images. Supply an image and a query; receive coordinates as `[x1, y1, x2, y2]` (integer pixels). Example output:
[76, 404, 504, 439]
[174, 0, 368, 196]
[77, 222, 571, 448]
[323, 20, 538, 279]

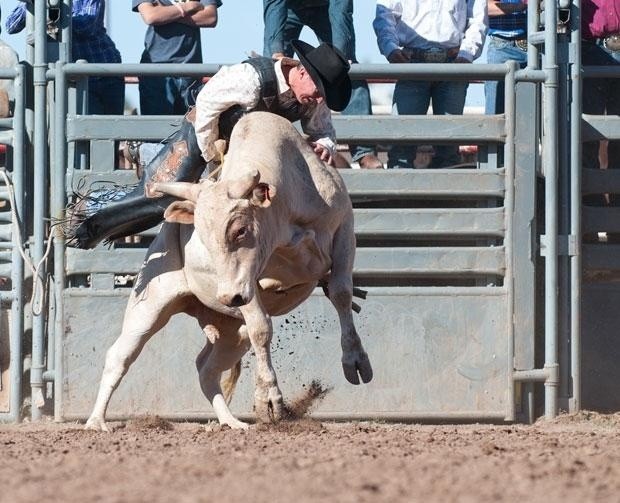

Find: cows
[85, 110, 373, 432]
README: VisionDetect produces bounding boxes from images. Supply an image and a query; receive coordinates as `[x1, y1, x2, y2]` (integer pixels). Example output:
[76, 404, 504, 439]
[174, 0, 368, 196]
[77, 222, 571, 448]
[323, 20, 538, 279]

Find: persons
[581, 0, 620, 244]
[483, 0, 527, 115]
[372, 0, 490, 168]
[48, 38, 352, 251]
[20, 0, 125, 170]
[262, 0, 383, 169]
[132, 0, 223, 115]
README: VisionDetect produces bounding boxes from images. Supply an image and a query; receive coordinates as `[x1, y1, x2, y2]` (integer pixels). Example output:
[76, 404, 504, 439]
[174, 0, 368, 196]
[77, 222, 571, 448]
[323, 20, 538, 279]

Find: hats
[290, 39, 351, 111]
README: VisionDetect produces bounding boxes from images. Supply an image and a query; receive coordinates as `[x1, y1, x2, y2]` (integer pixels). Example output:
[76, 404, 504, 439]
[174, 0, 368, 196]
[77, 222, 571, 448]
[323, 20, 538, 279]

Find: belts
[419, 46, 459, 66]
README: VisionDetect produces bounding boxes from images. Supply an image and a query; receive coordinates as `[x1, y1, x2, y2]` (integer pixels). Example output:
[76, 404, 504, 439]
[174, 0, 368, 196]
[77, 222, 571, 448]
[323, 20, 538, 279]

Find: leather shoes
[358, 155, 382, 168]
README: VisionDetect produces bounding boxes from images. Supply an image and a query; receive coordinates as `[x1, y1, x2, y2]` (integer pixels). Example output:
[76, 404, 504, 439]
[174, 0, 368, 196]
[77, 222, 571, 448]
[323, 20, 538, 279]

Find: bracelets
[174, 4, 185, 18]
